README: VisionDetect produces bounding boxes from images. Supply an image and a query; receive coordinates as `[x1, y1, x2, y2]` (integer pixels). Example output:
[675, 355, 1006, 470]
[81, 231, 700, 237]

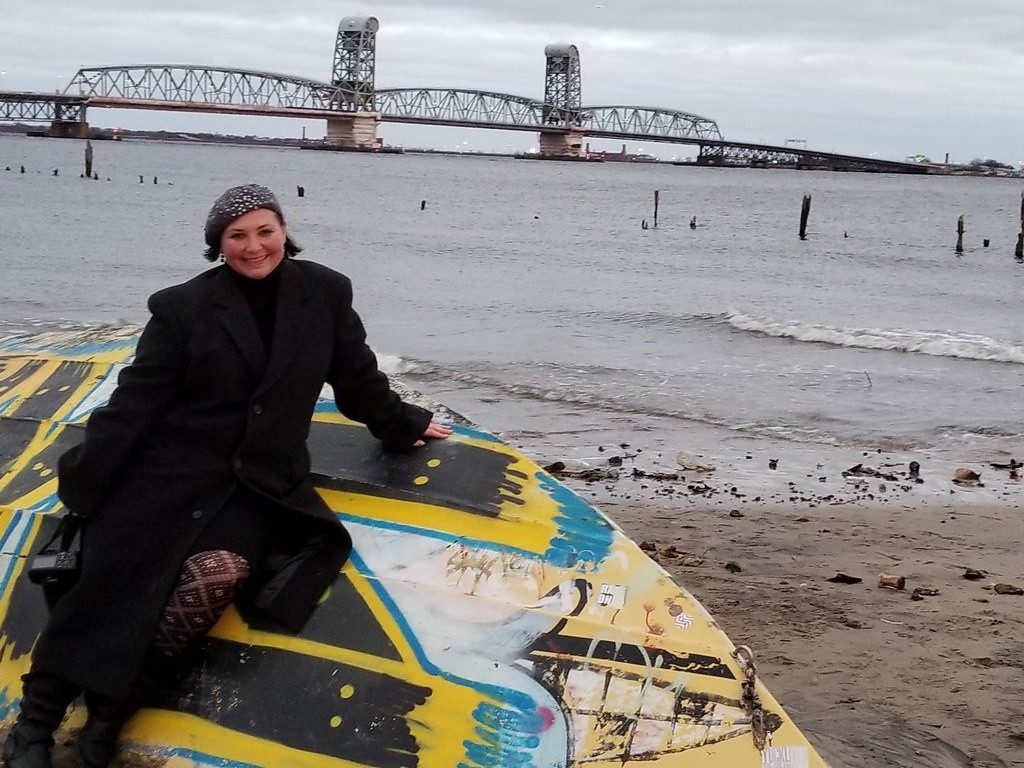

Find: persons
[0, 185, 453, 767]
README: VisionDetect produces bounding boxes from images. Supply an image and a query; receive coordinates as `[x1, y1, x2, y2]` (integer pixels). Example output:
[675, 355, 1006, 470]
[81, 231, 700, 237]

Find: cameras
[29, 553, 79, 614]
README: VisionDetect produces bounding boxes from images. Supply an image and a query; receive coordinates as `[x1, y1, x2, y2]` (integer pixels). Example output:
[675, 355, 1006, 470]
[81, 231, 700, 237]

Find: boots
[2, 664, 84, 768]
[72, 715, 122, 768]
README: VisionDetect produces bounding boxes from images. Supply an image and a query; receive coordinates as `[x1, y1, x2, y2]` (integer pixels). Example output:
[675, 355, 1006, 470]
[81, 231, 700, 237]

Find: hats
[205, 183, 282, 246]
